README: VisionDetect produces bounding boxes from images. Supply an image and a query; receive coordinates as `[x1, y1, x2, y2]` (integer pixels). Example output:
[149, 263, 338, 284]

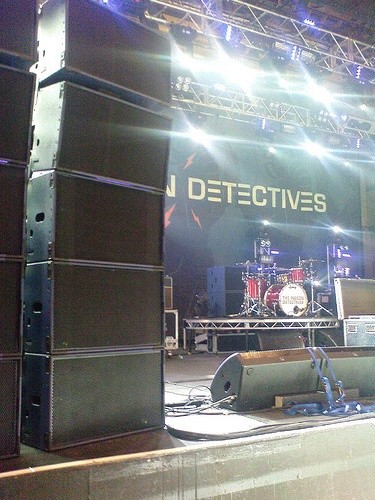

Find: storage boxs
[165, 310, 178, 359]
[333, 277, 375, 347]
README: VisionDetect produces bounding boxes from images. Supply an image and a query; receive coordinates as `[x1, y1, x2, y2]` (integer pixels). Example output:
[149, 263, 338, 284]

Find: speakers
[207, 265, 249, 317]
[333, 278, 375, 320]
[314, 347, 375, 397]
[209, 348, 321, 412]
[164, 276, 174, 309]
[257, 332, 305, 351]
[342, 319, 375, 347]
[1, 0, 173, 460]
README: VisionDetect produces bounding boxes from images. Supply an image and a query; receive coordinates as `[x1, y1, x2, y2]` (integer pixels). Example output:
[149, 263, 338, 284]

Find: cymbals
[301, 260, 319, 263]
[236, 263, 258, 266]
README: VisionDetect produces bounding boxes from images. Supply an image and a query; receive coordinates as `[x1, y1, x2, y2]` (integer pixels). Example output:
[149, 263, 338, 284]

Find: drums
[264, 282, 309, 316]
[289, 268, 305, 284]
[276, 274, 291, 284]
[246, 277, 267, 300]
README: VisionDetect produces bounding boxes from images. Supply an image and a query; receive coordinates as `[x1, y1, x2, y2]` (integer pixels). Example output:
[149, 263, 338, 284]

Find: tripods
[232, 263, 336, 317]
